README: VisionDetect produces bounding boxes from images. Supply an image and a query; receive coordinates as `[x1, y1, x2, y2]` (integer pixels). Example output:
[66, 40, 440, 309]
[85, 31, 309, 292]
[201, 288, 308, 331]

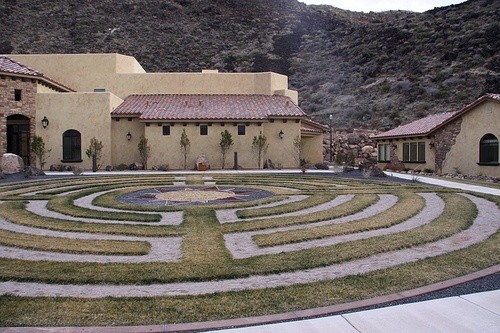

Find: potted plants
[195, 153, 209, 171]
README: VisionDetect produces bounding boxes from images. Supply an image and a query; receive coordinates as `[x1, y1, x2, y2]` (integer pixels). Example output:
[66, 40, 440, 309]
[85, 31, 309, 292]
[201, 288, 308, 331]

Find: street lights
[329, 114, 333, 161]
